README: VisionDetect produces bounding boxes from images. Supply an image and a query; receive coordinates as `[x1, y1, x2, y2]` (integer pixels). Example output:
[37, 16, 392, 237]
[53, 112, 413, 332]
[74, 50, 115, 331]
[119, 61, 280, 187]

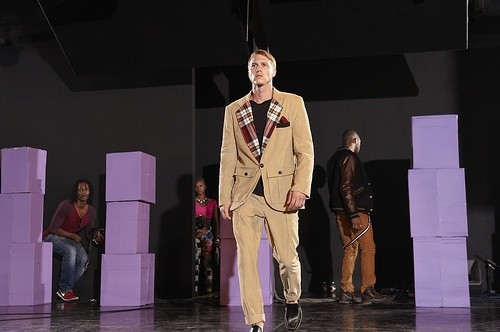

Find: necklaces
[195, 196, 208, 205]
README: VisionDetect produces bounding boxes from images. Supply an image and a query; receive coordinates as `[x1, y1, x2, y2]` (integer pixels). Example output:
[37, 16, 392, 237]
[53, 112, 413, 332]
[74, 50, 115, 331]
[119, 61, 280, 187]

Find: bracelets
[215, 238, 220, 241]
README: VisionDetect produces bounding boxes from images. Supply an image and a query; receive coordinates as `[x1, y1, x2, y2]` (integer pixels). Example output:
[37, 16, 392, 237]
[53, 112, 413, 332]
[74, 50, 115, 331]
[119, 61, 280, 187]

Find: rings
[359, 224, 362, 227]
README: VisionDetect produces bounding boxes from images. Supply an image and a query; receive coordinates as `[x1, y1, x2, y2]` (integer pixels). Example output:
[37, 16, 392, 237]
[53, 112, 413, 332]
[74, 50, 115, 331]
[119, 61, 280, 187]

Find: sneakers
[57, 288, 79, 301]
[339, 290, 373, 304]
[362, 286, 396, 302]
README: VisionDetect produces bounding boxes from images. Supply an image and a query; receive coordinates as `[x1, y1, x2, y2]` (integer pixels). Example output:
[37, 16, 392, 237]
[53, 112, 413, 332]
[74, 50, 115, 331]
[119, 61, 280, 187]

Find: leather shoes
[284, 299, 303, 331]
[251, 325, 264, 332]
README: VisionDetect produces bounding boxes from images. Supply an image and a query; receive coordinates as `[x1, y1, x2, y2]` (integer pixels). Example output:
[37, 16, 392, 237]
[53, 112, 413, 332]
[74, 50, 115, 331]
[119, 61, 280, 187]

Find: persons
[218, 49, 315, 332]
[193, 176, 221, 302]
[42, 178, 105, 302]
[326, 128, 395, 305]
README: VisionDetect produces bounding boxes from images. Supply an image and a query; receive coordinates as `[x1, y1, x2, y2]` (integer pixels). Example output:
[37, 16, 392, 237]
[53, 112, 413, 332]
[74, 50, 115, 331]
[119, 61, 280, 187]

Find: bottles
[330, 282, 337, 298]
[321, 282, 329, 298]
